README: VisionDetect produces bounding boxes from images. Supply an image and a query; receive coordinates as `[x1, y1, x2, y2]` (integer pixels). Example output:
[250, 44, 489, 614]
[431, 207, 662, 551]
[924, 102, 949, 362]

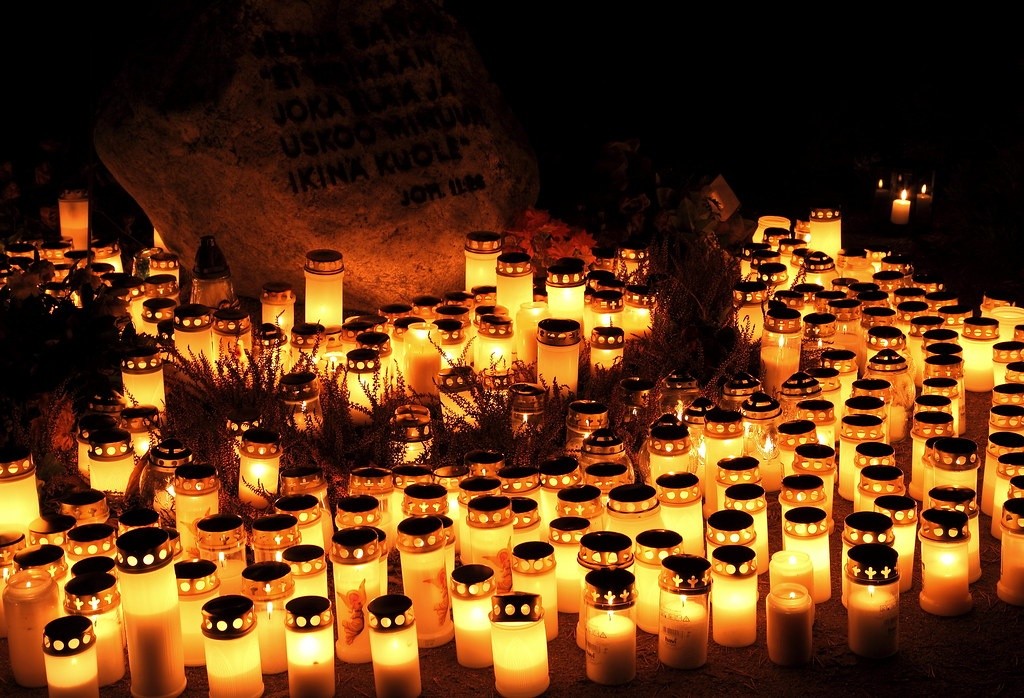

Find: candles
[0, 174, 1024, 698]
